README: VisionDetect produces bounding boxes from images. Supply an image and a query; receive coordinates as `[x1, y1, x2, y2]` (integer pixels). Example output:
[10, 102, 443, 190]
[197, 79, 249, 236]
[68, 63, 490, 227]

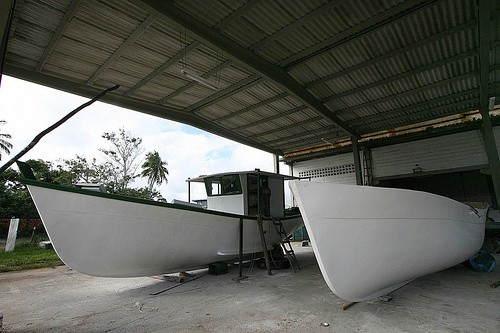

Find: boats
[288, 179, 491, 303]
[14, 160, 306, 278]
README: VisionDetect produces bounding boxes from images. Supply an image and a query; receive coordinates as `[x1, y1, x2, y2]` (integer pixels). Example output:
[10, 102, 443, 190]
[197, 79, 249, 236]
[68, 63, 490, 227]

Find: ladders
[271, 216, 301, 274]
